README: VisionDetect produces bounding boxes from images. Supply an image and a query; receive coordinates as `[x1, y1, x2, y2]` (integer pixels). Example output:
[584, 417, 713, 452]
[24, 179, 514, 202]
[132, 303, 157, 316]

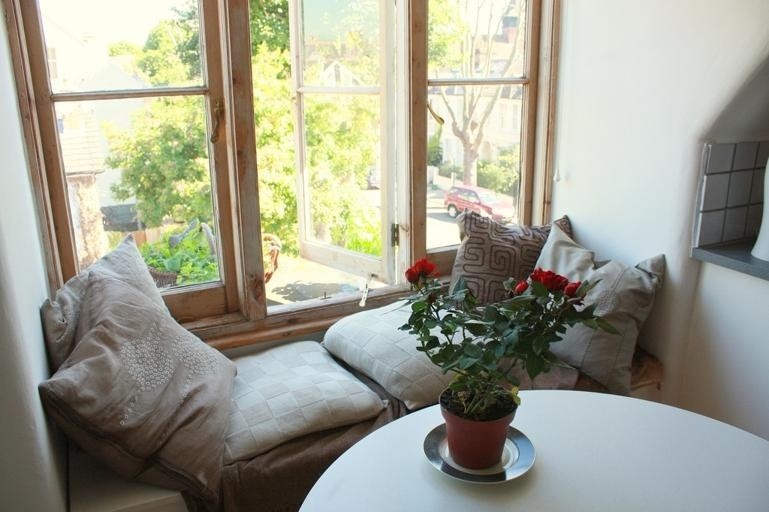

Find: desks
[295, 389, 769, 511]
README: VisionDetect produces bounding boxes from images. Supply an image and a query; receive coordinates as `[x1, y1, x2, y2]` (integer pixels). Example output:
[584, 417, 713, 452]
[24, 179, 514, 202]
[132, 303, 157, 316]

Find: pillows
[36, 233, 174, 370]
[520, 224, 667, 390]
[321, 292, 511, 410]
[36, 269, 240, 500]
[219, 339, 391, 465]
[449, 212, 571, 310]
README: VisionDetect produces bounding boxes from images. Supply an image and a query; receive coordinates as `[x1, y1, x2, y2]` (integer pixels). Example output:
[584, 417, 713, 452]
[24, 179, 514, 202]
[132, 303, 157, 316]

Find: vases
[437, 384, 518, 470]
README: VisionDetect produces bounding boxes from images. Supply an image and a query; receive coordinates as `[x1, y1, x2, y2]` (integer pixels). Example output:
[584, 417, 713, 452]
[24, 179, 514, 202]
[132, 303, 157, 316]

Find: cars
[444, 185, 514, 223]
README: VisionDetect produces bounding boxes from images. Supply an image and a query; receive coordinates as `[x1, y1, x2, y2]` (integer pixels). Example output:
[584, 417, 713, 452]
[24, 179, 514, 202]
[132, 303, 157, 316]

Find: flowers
[397, 258, 624, 412]
